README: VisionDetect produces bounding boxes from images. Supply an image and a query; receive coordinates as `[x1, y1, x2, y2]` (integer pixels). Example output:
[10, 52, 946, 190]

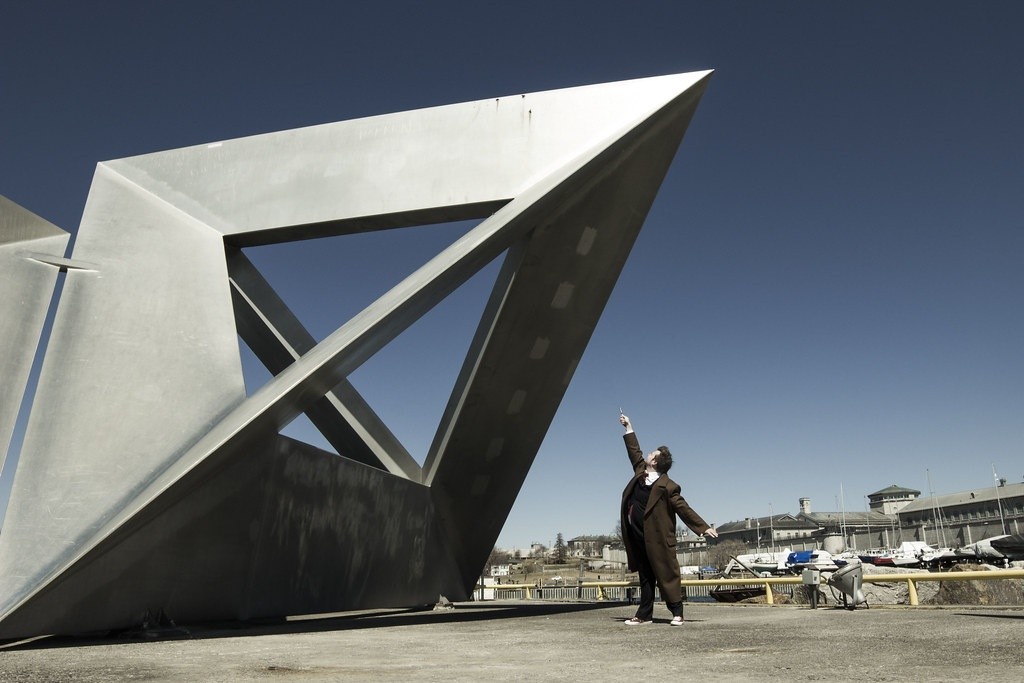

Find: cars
[552, 576, 562, 581]
[702, 566, 717, 571]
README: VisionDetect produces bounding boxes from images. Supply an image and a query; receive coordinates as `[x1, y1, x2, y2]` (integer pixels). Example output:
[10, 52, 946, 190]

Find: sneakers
[671, 616, 684, 625]
[625, 617, 652, 625]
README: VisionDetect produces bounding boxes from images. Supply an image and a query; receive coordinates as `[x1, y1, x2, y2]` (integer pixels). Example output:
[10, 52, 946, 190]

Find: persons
[620, 407, 720, 626]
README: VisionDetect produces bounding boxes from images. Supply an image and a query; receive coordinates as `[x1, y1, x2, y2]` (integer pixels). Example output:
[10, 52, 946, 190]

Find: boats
[785, 551, 812, 574]
[802, 550, 839, 570]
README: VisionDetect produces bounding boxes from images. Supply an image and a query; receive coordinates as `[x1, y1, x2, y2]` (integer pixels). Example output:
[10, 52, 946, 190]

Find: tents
[702, 566, 717, 575]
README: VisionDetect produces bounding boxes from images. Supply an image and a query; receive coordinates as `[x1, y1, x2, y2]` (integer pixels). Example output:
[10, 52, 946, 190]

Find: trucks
[679, 566, 701, 575]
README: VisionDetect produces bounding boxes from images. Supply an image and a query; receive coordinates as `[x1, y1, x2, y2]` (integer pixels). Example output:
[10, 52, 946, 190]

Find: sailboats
[920, 463, 1024, 569]
[725, 483, 935, 574]
